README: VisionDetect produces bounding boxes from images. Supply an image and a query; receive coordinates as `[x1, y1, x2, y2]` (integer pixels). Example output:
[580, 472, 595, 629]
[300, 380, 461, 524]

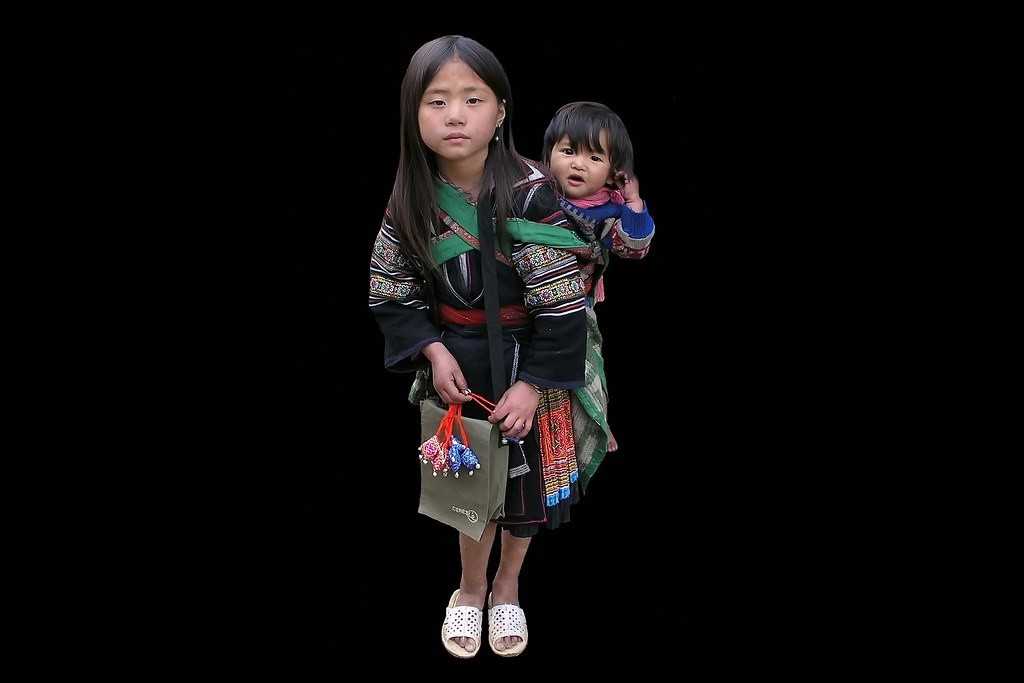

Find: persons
[539, 101, 653, 313]
[368, 37, 593, 659]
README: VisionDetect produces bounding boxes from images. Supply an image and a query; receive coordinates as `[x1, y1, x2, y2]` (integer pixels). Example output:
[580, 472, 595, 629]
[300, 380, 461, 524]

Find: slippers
[441, 589, 483, 659]
[487, 592, 528, 657]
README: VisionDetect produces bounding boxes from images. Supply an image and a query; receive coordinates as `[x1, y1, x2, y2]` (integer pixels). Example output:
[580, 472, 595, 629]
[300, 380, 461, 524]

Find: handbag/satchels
[417, 398, 510, 542]
